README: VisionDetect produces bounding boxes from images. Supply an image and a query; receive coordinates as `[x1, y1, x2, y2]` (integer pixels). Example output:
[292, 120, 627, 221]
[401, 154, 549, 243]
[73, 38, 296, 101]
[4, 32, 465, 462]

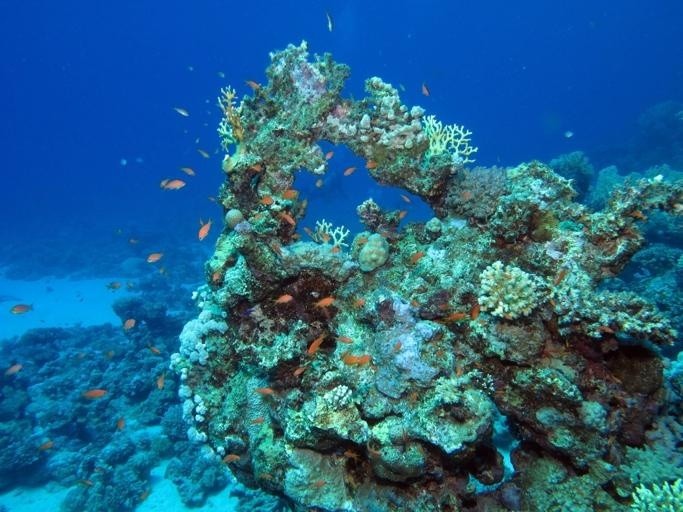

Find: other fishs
[124, 80, 480, 487]
